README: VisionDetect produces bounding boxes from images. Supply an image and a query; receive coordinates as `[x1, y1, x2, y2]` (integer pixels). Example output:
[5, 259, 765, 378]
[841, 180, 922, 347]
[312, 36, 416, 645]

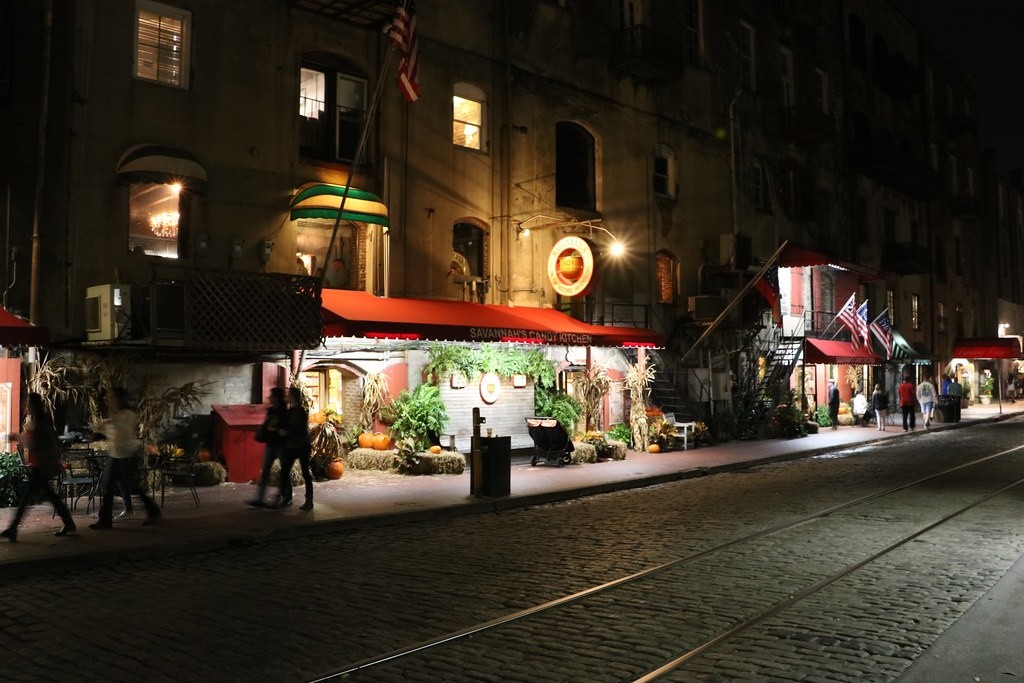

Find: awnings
[805, 338, 884, 366]
[116, 143, 207, 187]
[594, 325, 665, 351]
[952, 338, 1021, 359]
[0, 306, 50, 350]
[778, 242, 888, 283]
[299, 288, 623, 348]
[889, 331, 931, 366]
[290, 182, 389, 228]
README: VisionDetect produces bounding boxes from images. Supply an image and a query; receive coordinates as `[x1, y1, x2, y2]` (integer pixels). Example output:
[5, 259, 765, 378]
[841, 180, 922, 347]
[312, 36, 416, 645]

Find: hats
[828, 379, 835, 383]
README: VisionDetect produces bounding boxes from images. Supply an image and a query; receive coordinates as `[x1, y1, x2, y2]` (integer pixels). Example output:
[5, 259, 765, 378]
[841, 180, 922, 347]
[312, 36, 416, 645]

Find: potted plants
[977, 374, 995, 406]
[648, 417, 678, 452]
[960, 382, 972, 410]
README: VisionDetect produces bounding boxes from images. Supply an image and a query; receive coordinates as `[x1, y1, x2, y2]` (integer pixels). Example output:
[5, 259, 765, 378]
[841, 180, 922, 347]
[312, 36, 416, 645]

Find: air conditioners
[718, 231, 755, 270]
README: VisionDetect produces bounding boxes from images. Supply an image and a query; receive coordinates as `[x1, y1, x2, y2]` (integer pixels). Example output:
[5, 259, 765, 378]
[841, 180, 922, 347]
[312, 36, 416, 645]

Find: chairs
[662, 412, 696, 451]
[886, 403, 896, 426]
[11, 439, 204, 520]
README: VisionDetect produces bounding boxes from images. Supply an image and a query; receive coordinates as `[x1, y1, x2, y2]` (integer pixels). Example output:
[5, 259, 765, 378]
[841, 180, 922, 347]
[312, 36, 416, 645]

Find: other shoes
[89, 523, 112, 529]
[904, 430, 908, 432]
[923, 424, 930, 430]
[1012, 400, 1015, 403]
[141, 518, 161, 525]
[249, 501, 264, 508]
[55, 525, 76, 535]
[299, 504, 313, 511]
[0, 528, 17, 541]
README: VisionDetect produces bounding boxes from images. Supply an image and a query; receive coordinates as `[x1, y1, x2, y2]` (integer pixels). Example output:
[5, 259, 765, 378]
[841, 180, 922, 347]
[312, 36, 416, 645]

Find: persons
[86, 385, 162, 530]
[0, 392, 76, 542]
[898, 376, 916, 432]
[871, 384, 887, 431]
[852, 388, 872, 427]
[246, 387, 314, 510]
[827, 380, 839, 430]
[941, 374, 962, 419]
[915, 374, 939, 428]
[1008, 373, 1016, 403]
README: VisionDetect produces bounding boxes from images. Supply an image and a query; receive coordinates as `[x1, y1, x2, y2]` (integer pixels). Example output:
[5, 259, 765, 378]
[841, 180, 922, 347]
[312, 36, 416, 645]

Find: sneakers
[114, 512, 134, 520]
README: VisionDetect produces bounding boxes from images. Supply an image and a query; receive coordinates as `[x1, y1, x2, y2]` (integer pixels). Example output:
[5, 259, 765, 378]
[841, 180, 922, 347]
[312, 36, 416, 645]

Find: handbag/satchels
[255, 426, 270, 443]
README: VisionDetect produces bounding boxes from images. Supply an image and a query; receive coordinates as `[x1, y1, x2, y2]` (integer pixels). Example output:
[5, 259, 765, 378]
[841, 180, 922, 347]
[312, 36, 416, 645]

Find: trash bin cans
[470, 435, 511, 498]
[936, 395, 961, 422]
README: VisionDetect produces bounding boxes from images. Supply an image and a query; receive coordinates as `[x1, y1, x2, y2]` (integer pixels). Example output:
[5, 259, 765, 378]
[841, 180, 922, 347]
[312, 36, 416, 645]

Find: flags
[389, 0, 421, 104]
[869, 308, 892, 360]
[855, 301, 874, 354]
[837, 294, 860, 353]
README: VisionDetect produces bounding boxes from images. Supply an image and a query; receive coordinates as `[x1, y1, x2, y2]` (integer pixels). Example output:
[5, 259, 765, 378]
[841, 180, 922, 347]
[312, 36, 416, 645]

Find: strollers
[525, 417, 575, 468]
